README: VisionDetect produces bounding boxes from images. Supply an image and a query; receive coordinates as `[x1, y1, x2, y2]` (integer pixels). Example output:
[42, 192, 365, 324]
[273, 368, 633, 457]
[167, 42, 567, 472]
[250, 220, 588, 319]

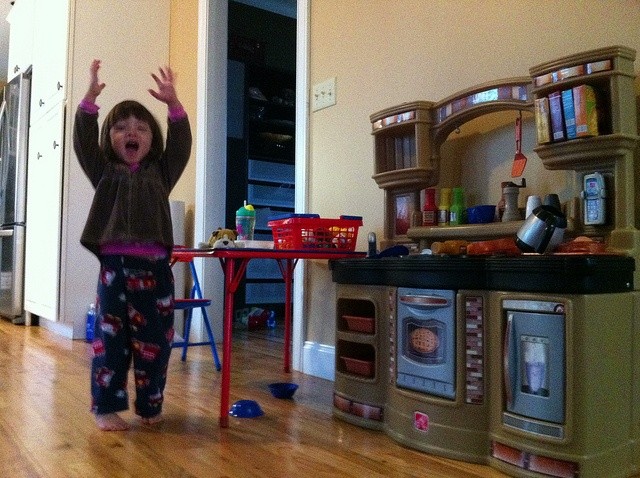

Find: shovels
[511, 116, 527, 178]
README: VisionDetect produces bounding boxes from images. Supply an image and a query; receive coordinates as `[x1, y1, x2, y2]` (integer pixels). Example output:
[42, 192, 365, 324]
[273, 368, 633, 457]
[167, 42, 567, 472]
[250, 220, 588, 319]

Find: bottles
[438, 188, 452, 227]
[235, 200, 256, 241]
[431, 240, 468, 254]
[86, 302, 99, 338]
[497, 178, 527, 222]
[450, 187, 464, 226]
[422, 187, 437, 226]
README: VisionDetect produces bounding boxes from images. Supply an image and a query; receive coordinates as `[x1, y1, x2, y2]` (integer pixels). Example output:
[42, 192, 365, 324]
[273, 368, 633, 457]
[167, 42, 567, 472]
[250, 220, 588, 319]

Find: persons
[72, 59, 193, 432]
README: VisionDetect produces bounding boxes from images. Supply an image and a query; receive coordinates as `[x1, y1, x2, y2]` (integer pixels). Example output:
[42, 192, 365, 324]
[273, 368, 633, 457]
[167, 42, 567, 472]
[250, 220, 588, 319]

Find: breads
[561, 235, 608, 255]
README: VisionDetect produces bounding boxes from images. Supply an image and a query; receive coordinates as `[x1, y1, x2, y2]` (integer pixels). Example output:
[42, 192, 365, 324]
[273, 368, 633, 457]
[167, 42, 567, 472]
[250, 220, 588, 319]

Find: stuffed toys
[208, 227, 245, 249]
